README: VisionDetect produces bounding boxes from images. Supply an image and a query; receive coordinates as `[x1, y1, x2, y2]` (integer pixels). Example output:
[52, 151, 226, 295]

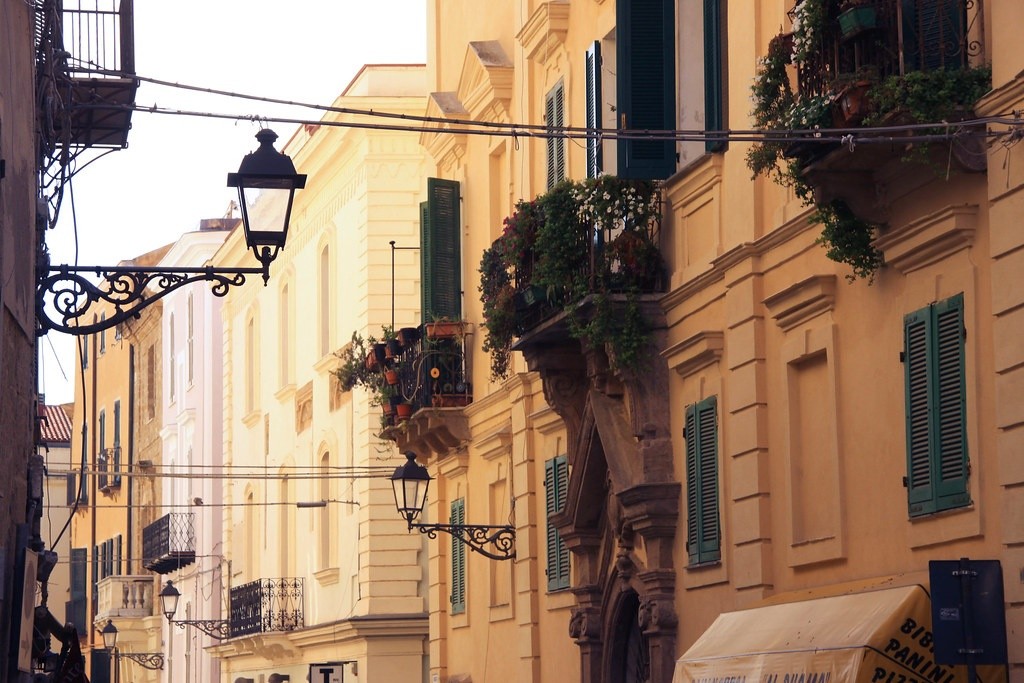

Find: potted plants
[330, 310, 473, 425]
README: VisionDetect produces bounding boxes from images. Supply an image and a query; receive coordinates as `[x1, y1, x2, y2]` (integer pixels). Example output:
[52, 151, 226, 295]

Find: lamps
[389, 452, 517, 561]
[158, 580, 231, 639]
[34, 129, 306, 338]
[101, 619, 165, 671]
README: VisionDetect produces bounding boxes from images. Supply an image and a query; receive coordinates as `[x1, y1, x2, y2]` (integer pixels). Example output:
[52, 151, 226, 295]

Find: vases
[834, 79, 881, 122]
[836, 6, 877, 35]
[819, 104, 851, 145]
[515, 285, 544, 312]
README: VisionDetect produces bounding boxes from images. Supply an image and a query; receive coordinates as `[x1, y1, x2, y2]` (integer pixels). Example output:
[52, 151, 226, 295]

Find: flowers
[742, 0, 993, 285]
[477, 174, 665, 383]
